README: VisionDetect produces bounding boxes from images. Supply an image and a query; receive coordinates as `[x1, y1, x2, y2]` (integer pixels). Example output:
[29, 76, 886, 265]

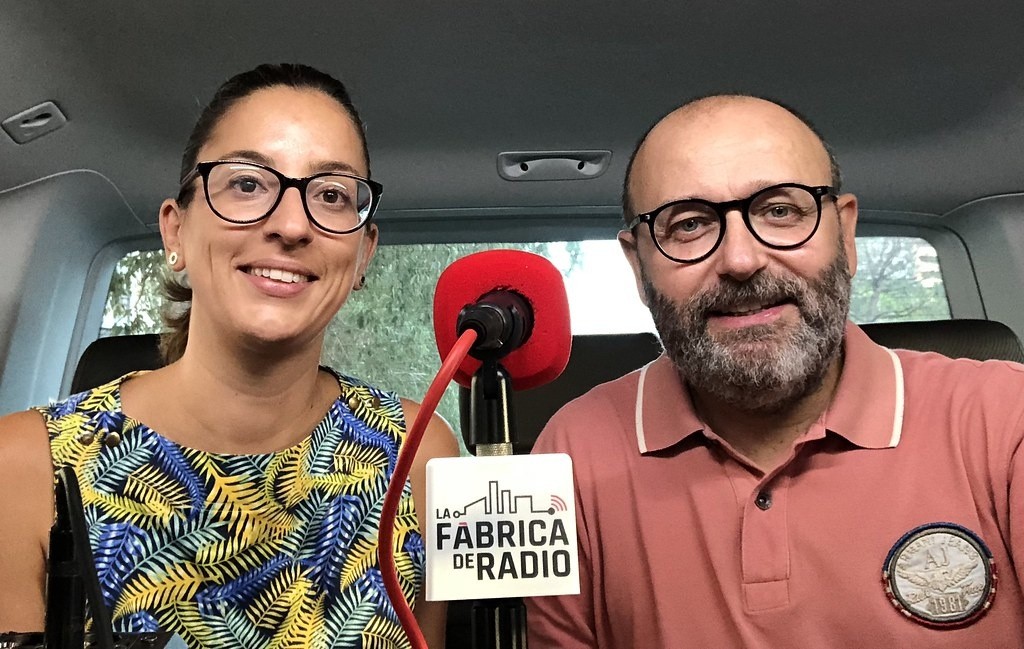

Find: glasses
[180, 156, 383, 235]
[625, 181, 838, 264]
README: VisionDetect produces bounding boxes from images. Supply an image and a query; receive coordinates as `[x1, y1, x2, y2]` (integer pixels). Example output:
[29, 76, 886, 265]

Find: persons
[516, 91, 1023, 648]
[0, 62, 459, 649]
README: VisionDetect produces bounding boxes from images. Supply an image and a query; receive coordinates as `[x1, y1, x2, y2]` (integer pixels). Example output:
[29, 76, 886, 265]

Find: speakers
[433, 251, 572, 392]
